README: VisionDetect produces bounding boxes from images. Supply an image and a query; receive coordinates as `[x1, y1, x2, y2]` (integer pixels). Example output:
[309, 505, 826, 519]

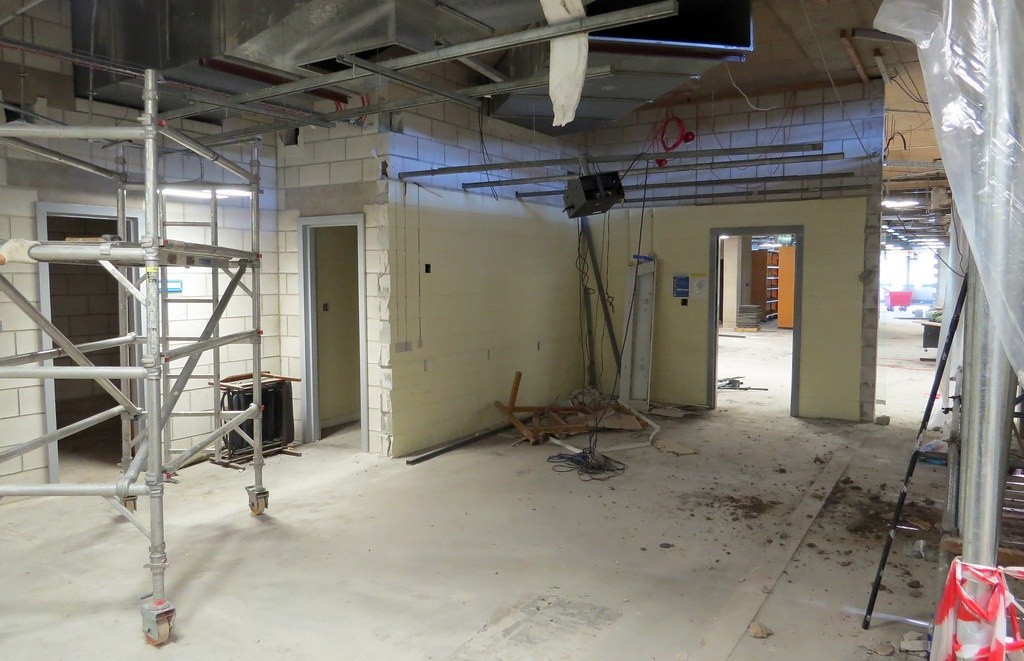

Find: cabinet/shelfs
[751, 249, 779, 322]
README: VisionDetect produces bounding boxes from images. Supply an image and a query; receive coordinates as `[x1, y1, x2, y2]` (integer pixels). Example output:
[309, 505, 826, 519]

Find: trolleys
[880, 287, 912, 311]
